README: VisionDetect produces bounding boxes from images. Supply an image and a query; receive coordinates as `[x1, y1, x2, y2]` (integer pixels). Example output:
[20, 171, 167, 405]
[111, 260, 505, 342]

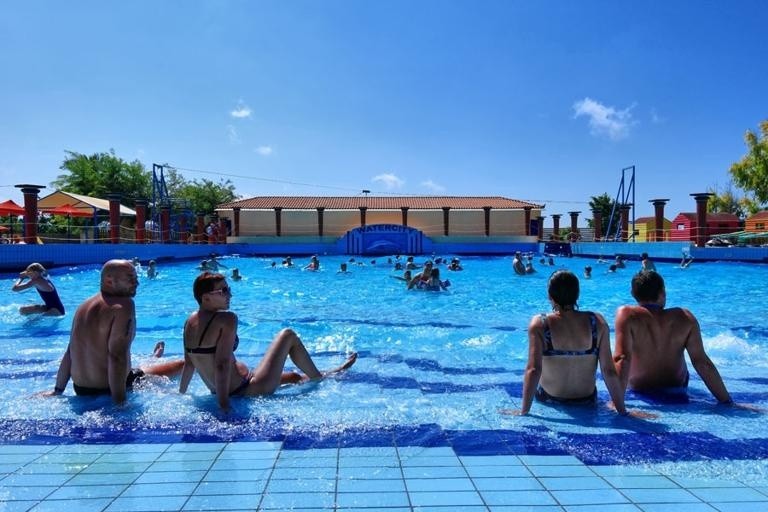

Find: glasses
[210, 286, 231, 295]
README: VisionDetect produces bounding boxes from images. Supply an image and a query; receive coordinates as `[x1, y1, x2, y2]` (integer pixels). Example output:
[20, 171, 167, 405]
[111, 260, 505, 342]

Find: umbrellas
[0, 200, 93, 244]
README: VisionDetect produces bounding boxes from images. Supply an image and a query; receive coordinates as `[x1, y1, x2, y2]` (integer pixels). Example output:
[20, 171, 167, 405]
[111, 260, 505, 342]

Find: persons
[603, 269, 767, 412]
[584, 251, 627, 279]
[269, 254, 377, 275]
[388, 253, 464, 293]
[512, 250, 565, 276]
[206, 222, 221, 244]
[145, 219, 160, 243]
[125, 256, 159, 280]
[11, 263, 64, 323]
[195, 252, 241, 283]
[495, 270, 658, 418]
[29, 259, 196, 412]
[640, 253, 656, 273]
[156, 272, 357, 415]
[679, 249, 695, 268]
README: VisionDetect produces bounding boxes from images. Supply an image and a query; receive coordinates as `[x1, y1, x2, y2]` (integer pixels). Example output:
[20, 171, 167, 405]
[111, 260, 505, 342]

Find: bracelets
[719, 396, 732, 406]
[55, 386, 65, 393]
